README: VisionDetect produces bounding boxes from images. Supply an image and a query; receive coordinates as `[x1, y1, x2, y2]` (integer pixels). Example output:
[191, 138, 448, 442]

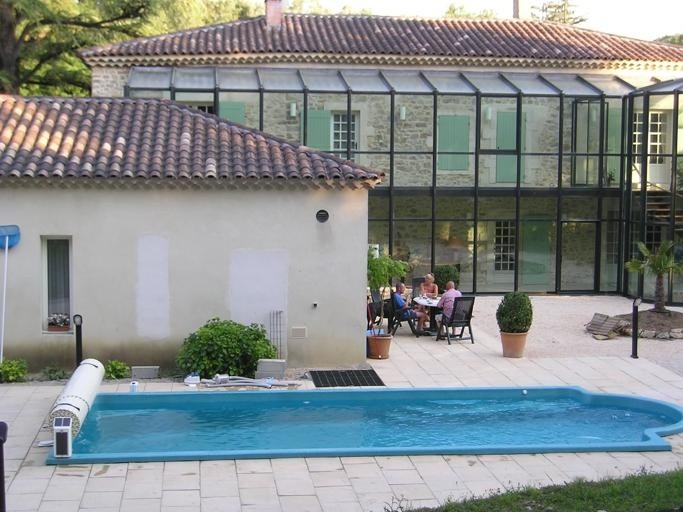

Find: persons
[392, 282, 430, 336]
[434, 281, 464, 340]
[419, 273, 438, 330]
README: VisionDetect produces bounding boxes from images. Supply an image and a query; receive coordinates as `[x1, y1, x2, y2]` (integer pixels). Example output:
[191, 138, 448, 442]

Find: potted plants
[367, 245, 410, 359]
[496, 291, 532, 358]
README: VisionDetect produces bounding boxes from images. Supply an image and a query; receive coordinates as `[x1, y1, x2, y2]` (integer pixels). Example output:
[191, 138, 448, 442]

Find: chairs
[370, 277, 474, 346]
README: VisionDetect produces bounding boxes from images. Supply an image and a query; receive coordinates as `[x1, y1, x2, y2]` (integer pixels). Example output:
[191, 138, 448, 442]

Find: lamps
[290, 103, 296, 117]
[485, 107, 492, 121]
[592, 108, 596, 123]
[400, 106, 406, 120]
